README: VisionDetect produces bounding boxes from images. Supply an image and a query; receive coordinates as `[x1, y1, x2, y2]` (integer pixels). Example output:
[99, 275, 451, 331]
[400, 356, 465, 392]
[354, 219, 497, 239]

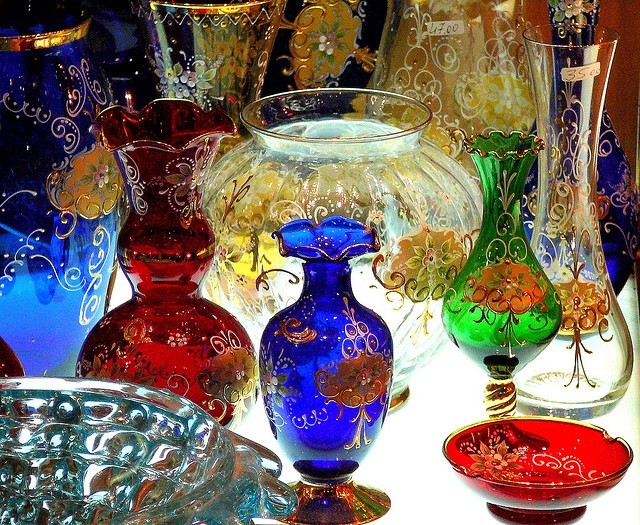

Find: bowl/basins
[443, 415, 634, 525]
